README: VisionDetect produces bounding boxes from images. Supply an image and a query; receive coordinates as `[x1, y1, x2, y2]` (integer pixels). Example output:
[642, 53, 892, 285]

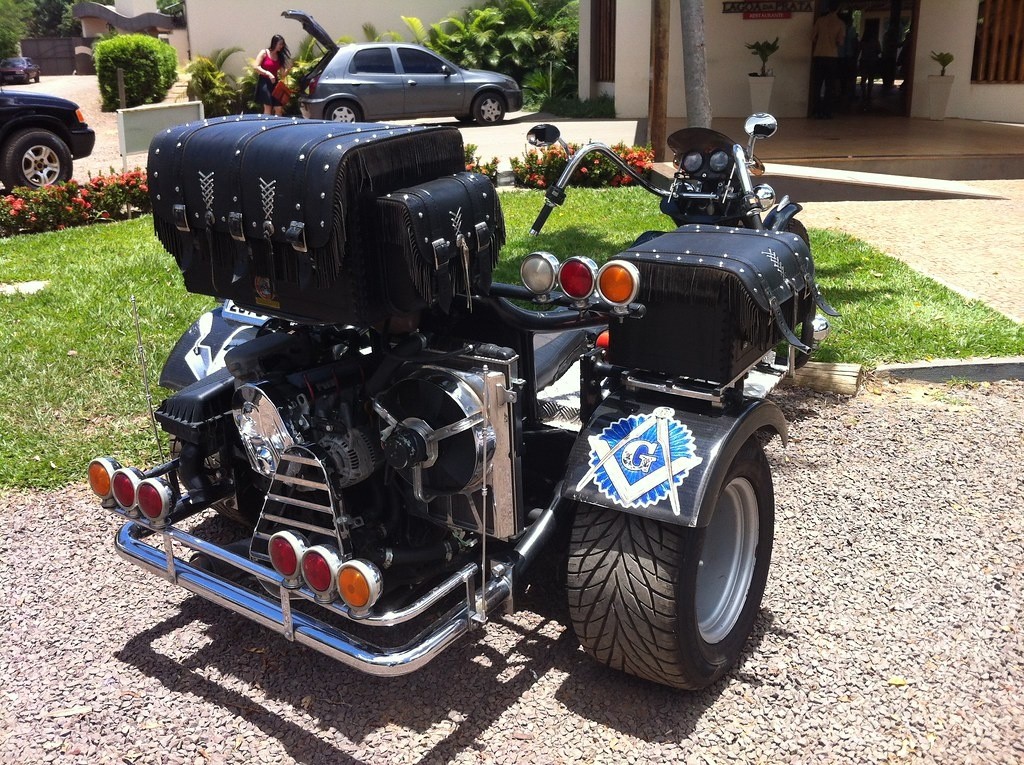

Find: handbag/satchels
[271, 72, 299, 105]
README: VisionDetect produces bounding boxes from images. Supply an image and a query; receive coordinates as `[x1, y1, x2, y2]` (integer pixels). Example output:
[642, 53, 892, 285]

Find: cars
[0, 87, 95, 199]
[0, 57, 40, 85]
[280, 9, 523, 128]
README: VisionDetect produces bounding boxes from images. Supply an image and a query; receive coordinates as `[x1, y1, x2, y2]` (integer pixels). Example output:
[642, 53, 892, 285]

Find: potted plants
[929, 52, 956, 120]
[746, 37, 781, 113]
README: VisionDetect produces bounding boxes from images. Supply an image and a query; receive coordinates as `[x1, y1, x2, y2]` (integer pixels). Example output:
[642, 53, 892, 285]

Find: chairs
[447, 283, 608, 424]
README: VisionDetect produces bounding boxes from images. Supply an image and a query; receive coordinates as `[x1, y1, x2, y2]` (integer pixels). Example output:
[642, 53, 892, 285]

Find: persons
[810, 0, 846, 118]
[253, 35, 293, 116]
[842, 23, 911, 98]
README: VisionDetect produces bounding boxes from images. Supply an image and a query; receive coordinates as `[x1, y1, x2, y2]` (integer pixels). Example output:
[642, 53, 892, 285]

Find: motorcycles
[89, 113, 842, 691]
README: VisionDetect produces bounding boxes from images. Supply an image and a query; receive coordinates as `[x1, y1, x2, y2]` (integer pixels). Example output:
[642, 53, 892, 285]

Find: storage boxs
[147, 119, 841, 390]
